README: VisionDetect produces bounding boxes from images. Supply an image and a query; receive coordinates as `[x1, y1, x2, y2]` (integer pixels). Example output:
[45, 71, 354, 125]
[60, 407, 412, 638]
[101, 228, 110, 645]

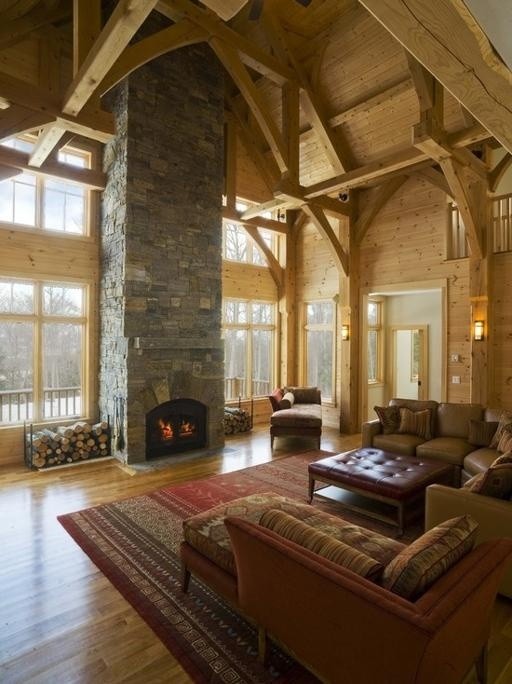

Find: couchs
[268, 386, 323, 450]
[362, 397, 512, 539]
[179, 487, 512, 684]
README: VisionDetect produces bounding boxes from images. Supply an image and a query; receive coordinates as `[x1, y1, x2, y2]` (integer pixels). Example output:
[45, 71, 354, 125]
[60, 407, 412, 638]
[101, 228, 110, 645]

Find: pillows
[382, 515, 479, 599]
[373, 404, 407, 434]
[278, 392, 295, 409]
[283, 386, 320, 404]
[462, 419, 512, 494]
[397, 407, 432, 441]
[260, 509, 384, 584]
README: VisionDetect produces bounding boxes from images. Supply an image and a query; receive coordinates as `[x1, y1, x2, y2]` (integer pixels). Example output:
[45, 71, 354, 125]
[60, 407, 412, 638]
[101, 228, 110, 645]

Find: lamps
[341, 324, 349, 340]
[474, 320, 484, 341]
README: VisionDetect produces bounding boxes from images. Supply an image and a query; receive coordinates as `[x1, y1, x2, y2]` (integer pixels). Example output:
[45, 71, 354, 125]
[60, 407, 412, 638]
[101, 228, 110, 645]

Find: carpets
[56, 448, 512, 684]
